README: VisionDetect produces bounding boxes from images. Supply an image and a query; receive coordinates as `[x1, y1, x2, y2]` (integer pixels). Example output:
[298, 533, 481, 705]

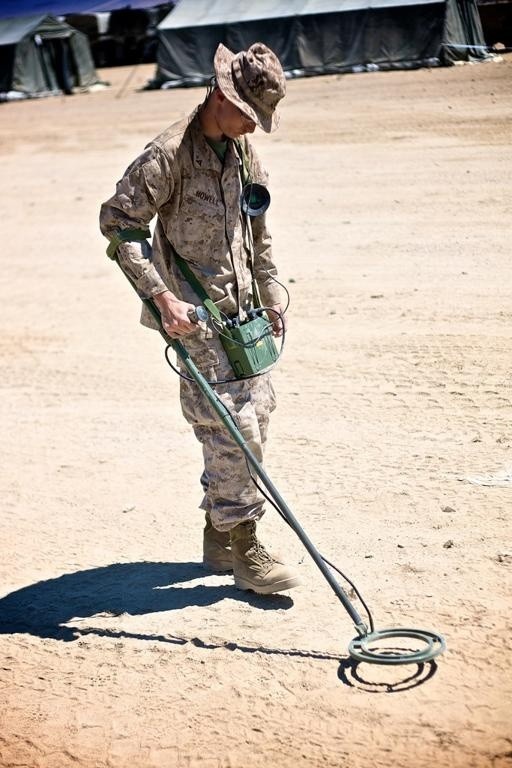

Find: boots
[203, 512, 233, 573]
[230, 519, 301, 595]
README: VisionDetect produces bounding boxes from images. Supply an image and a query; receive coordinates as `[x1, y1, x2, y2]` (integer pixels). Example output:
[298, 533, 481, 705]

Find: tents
[1, 14, 112, 99]
[479, 1, 512, 54]
[138, 1, 496, 92]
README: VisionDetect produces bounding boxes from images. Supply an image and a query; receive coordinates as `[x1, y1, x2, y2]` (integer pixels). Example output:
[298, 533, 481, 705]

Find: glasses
[236, 107, 256, 130]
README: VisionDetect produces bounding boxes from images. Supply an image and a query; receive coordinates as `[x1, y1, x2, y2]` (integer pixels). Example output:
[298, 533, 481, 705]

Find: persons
[100, 42, 300, 604]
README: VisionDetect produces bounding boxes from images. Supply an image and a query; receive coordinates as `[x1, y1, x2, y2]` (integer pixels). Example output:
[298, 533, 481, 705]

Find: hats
[213, 42, 286, 133]
